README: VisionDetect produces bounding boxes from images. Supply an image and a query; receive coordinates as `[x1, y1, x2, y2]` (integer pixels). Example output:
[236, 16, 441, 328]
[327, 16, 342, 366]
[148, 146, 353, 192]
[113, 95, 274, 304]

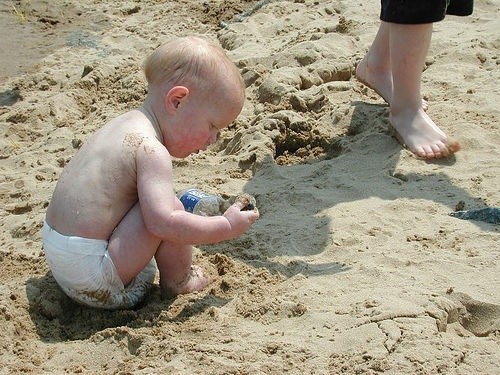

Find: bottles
[177, 189, 230, 217]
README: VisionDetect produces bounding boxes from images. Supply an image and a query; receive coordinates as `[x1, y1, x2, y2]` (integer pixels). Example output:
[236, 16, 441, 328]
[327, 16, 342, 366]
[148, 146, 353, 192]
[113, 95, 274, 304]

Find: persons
[356, 0, 474, 159]
[42, 35, 260, 311]
